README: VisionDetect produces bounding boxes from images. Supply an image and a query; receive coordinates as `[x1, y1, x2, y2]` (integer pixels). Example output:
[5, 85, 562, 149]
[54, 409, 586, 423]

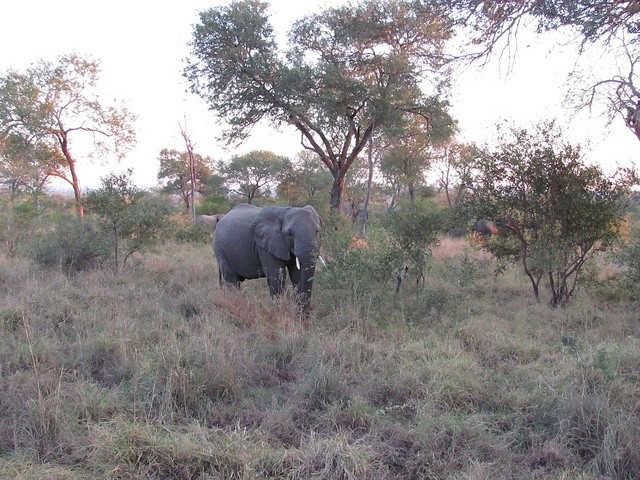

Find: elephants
[471, 217, 499, 239]
[212, 203, 326, 321]
[196, 214, 226, 233]
